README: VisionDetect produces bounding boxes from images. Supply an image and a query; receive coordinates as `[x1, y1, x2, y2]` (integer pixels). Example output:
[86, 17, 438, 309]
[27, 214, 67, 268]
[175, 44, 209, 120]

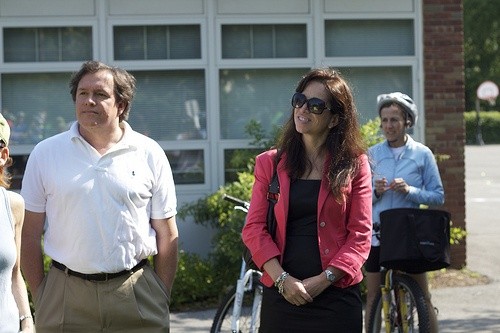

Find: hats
[0, 113, 10, 147]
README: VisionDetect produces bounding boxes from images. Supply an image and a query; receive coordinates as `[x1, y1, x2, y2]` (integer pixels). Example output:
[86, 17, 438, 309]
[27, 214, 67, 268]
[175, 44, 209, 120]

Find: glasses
[291, 92, 331, 116]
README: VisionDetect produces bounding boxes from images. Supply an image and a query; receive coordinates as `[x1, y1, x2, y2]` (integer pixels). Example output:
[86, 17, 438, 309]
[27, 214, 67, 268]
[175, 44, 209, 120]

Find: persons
[241, 69, 372, 333]
[363, 92, 445, 333]
[0, 114, 35, 333]
[20, 63, 179, 333]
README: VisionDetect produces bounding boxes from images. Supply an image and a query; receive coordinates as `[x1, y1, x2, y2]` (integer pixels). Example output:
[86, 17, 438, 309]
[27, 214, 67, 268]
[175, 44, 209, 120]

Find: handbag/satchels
[379, 208, 450, 272]
[238, 147, 282, 270]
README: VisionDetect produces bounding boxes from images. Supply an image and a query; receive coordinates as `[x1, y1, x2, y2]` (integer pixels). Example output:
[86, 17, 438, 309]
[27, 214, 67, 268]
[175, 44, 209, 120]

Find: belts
[52, 259, 146, 282]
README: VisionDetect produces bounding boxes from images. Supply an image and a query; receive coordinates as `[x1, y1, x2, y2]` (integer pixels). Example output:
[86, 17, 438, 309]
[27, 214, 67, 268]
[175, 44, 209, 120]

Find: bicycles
[372, 207, 451, 333]
[210, 194, 262, 333]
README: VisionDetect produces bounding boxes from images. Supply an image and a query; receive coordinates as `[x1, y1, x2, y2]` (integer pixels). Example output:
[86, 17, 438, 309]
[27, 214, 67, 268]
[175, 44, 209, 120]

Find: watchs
[20, 315, 32, 321]
[325, 269, 336, 284]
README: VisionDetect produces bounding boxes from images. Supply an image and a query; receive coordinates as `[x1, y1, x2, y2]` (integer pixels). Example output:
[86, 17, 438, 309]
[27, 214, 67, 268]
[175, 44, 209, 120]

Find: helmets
[378, 92, 417, 127]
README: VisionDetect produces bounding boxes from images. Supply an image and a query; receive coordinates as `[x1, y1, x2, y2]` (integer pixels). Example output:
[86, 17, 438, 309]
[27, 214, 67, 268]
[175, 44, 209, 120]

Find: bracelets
[274, 272, 289, 294]
